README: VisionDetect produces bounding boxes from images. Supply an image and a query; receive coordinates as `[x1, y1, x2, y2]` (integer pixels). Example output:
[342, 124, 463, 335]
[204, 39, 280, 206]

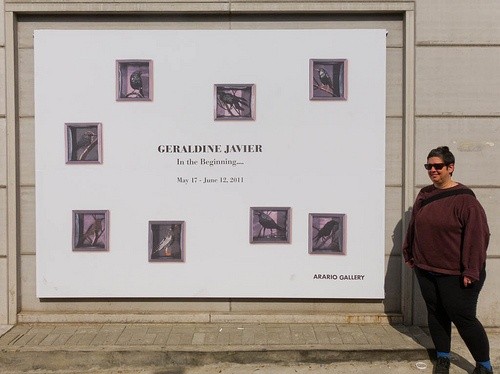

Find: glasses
[424, 163, 445, 170]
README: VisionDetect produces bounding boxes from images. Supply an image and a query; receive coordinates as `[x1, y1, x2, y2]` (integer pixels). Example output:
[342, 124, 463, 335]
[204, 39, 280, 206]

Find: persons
[403, 146, 493, 374]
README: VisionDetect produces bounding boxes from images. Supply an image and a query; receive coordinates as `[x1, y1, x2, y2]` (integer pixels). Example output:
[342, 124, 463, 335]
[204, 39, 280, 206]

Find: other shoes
[471, 363, 493, 374]
[434, 356, 450, 374]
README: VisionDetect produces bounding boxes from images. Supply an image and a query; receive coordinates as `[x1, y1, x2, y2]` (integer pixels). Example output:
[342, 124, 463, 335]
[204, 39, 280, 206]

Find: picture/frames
[308, 213, 347, 256]
[115, 59, 154, 101]
[309, 58, 347, 102]
[214, 84, 256, 121]
[72, 209, 109, 253]
[249, 206, 292, 244]
[65, 123, 103, 164]
[148, 220, 185, 262]
